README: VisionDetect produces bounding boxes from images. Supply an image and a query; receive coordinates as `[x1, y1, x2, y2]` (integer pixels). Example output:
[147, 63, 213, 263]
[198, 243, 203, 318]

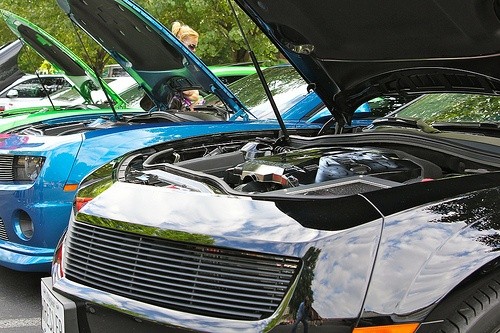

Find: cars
[1, 1, 395, 274]
[40, 0, 500, 332]
[0, 75, 73, 112]
[0, 8, 268, 133]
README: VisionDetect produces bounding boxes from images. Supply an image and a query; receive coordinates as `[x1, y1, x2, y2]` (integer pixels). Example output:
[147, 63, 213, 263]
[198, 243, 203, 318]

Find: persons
[171, 20, 204, 100]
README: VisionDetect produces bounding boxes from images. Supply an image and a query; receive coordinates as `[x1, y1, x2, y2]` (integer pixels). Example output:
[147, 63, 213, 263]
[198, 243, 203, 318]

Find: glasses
[182, 40, 198, 50]
